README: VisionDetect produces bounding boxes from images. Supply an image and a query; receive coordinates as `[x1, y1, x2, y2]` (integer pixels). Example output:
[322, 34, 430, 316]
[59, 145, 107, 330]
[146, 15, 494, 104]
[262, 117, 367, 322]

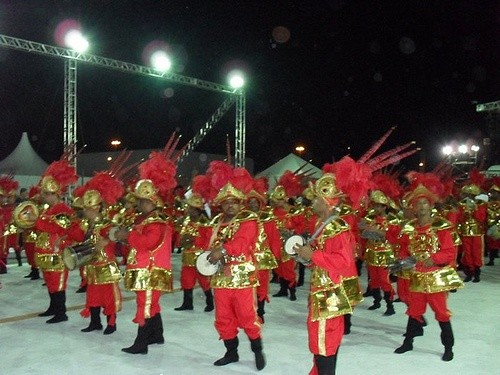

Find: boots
[39, 295, 55, 316]
[121, 318, 152, 354]
[272, 278, 289, 297]
[174, 289, 193, 310]
[31, 269, 39, 280]
[290, 288, 296, 301]
[148, 313, 164, 345]
[298, 263, 305, 285]
[24, 268, 35, 278]
[314, 355, 336, 375]
[104, 314, 116, 334]
[344, 249, 495, 361]
[46, 291, 68, 323]
[204, 289, 214, 312]
[257, 301, 265, 323]
[81, 306, 102, 332]
[250, 338, 264, 370]
[214, 336, 239, 366]
[177, 248, 181, 253]
[17, 258, 22, 266]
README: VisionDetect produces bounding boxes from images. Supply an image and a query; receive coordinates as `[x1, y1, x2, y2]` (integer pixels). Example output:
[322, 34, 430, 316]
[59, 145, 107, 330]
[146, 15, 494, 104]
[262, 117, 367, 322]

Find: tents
[0, 132, 52, 193]
[256, 153, 324, 186]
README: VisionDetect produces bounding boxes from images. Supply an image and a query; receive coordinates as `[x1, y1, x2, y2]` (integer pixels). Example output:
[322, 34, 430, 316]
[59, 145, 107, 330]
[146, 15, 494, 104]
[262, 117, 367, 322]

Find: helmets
[0, 125, 500, 213]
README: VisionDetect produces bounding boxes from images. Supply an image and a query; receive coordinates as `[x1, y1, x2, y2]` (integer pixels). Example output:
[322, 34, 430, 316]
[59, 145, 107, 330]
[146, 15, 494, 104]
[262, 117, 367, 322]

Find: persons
[394, 198, 464, 361]
[207, 200, 266, 370]
[293, 197, 364, 375]
[114, 197, 174, 354]
[0, 177, 500, 336]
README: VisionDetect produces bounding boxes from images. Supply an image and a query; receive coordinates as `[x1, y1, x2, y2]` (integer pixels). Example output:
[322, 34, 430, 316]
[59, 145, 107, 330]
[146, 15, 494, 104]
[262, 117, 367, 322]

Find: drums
[61, 241, 97, 271]
[12, 200, 41, 229]
[107, 225, 128, 245]
[194, 250, 221, 277]
[284, 235, 317, 269]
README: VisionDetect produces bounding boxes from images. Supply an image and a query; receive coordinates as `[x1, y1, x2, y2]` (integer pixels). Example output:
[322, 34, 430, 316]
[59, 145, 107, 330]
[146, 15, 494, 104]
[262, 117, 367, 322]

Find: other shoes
[270, 277, 276, 283]
[76, 288, 85, 293]
